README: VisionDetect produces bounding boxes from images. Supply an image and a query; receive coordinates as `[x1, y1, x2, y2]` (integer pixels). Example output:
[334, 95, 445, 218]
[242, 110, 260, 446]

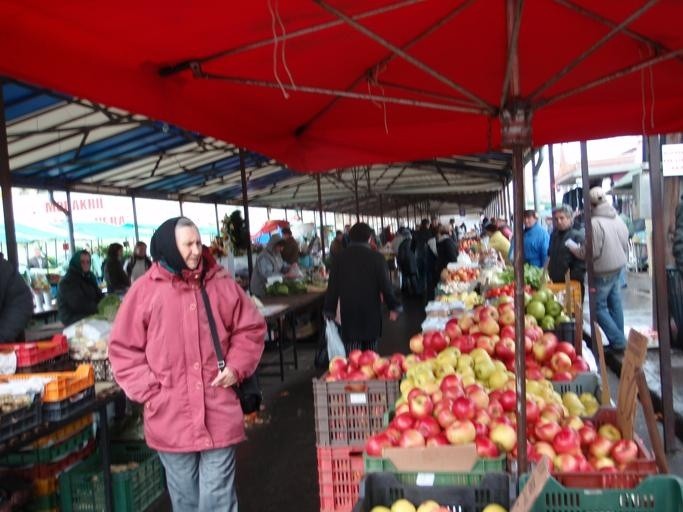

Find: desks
[259, 293, 326, 381]
[2, 382, 124, 512]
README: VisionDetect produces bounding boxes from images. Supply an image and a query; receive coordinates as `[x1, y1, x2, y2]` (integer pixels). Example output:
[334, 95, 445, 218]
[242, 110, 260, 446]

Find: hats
[588, 186, 606, 204]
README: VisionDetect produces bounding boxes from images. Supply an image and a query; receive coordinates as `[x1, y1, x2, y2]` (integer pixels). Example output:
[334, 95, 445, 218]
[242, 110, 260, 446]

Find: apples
[325, 266, 638, 474]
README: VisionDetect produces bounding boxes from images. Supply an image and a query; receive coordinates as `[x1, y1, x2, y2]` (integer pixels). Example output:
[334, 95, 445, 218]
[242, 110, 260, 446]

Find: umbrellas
[1, 0, 683, 511]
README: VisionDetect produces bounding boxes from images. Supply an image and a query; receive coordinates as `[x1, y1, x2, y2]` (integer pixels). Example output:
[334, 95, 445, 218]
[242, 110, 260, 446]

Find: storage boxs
[59, 439, 165, 512]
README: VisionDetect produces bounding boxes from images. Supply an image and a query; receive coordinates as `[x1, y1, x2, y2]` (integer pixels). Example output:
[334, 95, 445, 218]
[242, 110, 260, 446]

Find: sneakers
[604, 343, 629, 358]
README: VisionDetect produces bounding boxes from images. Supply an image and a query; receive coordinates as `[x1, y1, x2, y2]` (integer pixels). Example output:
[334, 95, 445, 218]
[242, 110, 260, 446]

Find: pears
[371, 498, 508, 512]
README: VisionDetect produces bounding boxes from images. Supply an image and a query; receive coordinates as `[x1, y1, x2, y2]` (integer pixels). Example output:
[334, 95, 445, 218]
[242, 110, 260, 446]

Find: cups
[565, 238, 579, 251]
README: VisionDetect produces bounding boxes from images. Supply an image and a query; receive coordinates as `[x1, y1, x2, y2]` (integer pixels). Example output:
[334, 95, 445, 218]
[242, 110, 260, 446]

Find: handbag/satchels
[237, 373, 263, 414]
